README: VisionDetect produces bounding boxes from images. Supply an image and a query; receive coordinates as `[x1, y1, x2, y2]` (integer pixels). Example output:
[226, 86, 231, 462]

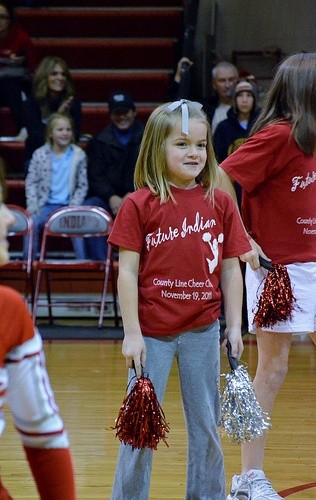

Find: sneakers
[226, 470, 283, 500]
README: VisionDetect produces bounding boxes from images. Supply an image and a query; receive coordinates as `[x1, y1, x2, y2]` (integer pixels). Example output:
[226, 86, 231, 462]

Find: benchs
[0, 0, 185, 205]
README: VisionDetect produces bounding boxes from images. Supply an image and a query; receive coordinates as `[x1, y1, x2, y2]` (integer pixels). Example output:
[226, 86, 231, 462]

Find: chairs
[33, 205, 119, 329]
[0, 204, 32, 300]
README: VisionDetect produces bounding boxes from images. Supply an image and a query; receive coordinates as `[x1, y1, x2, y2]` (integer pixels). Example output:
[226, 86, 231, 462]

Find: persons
[0, 5, 262, 261]
[218, 52, 316, 500]
[0, 170, 77, 500]
[107, 99, 253, 500]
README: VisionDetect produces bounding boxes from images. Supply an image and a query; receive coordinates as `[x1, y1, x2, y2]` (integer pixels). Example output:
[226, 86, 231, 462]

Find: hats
[108, 93, 137, 115]
[231, 79, 259, 110]
